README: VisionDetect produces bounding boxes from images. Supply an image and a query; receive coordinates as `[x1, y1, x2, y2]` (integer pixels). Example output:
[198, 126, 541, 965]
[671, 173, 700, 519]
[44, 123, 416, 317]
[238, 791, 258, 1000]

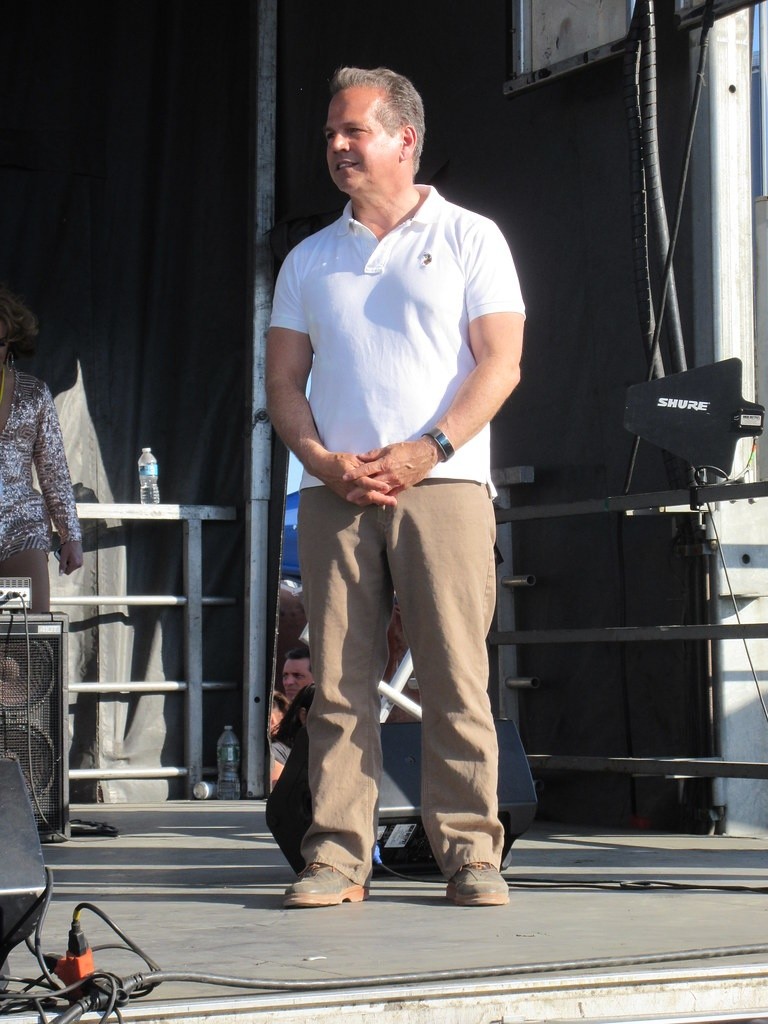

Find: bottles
[138, 447, 160, 504]
[216, 726, 241, 800]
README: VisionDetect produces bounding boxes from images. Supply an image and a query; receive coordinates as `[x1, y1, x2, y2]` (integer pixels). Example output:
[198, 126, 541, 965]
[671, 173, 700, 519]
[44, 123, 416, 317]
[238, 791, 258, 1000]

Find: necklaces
[0, 368, 5, 401]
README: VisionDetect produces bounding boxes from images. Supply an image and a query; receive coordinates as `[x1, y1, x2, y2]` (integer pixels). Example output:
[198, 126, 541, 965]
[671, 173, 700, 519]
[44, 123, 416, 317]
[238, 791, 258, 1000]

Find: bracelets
[421, 428, 455, 462]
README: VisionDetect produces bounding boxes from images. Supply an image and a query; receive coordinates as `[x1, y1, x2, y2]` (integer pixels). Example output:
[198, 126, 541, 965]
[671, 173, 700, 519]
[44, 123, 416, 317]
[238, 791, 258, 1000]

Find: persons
[268, 648, 317, 793]
[264, 66, 527, 907]
[0, 283, 82, 612]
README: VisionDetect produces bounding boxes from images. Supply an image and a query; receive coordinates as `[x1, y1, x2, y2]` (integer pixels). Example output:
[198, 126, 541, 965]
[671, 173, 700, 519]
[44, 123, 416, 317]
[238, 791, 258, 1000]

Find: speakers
[263, 716, 540, 883]
[0, 610, 70, 842]
[0, 759, 48, 954]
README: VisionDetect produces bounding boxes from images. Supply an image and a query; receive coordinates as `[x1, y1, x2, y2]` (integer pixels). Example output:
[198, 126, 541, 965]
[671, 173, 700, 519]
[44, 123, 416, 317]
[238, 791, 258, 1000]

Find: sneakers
[284, 856, 370, 906]
[445, 859, 508, 899]
